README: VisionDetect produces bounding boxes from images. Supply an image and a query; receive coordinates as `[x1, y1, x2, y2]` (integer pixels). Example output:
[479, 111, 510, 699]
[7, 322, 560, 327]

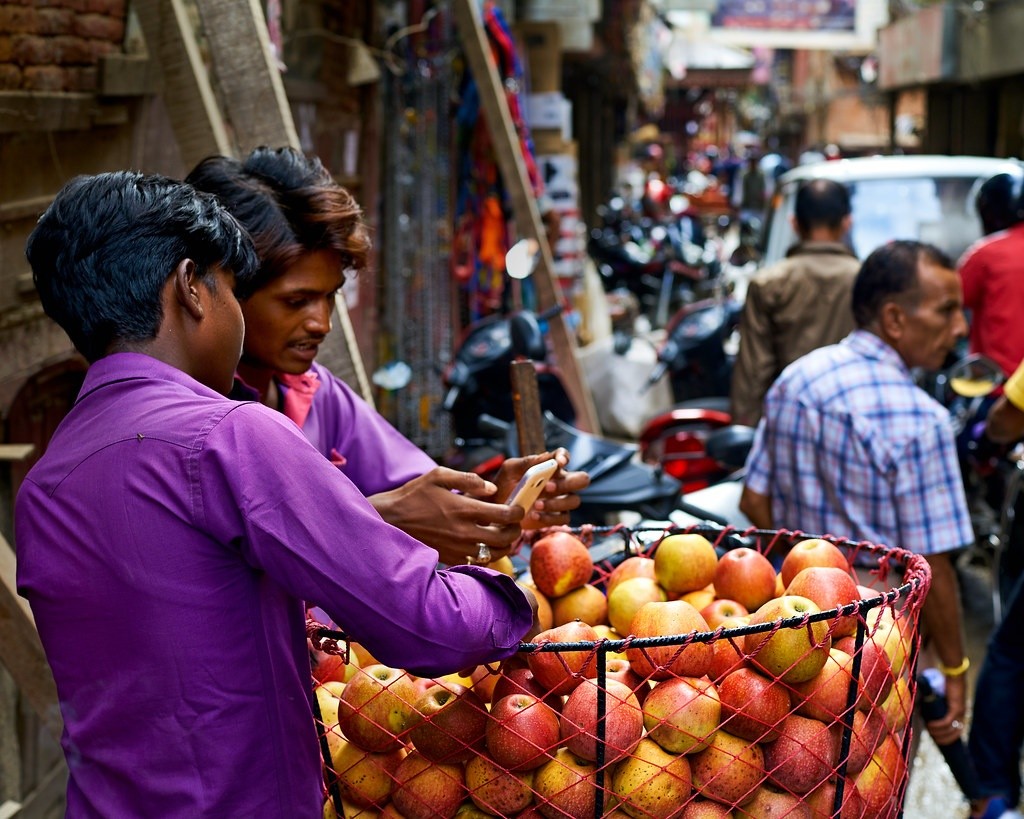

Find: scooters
[372, 146, 1024, 634]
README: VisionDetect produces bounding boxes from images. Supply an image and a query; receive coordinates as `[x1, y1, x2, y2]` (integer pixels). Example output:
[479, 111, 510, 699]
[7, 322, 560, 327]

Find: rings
[952, 721, 963, 730]
[476, 543, 492, 562]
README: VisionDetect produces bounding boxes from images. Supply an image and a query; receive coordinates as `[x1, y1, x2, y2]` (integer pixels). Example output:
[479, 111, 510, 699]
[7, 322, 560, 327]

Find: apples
[307, 532, 913, 819]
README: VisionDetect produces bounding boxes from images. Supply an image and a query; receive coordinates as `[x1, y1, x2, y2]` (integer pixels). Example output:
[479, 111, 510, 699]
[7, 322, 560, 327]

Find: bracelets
[938, 657, 970, 674]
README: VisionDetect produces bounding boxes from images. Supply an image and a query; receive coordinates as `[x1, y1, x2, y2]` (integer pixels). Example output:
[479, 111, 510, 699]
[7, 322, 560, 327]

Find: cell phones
[489, 458, 558, 528]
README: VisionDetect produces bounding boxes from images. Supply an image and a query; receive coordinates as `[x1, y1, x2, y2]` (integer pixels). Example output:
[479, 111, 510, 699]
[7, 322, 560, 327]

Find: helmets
[975, 171, 1024, 234]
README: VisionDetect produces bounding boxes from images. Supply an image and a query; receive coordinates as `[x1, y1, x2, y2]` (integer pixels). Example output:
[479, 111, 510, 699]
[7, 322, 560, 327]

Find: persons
[187, 146, 590, 638]
[732, 179, 864, 429]
[964, 356, 1024, 819]
[14, 169, 542, 818]
[956, 166, 1024, 516]
[640, 134, 827, 218]
[739, 241, 974, 745]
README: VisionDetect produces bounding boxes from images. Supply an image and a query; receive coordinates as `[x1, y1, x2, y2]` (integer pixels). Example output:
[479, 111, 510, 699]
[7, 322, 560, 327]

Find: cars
[728, 153, 1024, 356]
